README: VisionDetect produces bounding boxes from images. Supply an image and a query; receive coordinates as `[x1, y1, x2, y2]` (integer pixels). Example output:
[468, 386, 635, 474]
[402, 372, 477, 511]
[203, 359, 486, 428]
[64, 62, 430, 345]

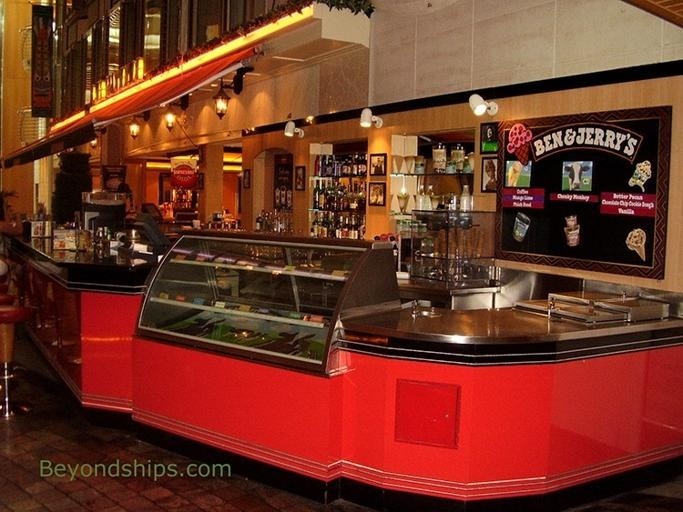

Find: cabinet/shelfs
[305, 175, 367, 213]
[410, 208, 497, 289]
[128, 231, 402, 506]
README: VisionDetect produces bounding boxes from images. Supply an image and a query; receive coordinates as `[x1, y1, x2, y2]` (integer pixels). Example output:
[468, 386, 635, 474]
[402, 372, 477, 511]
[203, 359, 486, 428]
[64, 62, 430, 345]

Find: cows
[563, 162, 591, 191]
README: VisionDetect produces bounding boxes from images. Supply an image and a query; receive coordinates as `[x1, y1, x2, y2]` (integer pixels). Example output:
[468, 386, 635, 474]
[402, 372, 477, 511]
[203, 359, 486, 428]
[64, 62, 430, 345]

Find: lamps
[359, 108, 384, 130]
[468, 94, 499, 116]
[127, 77, 236, 140]
[283, 121, 305, 139]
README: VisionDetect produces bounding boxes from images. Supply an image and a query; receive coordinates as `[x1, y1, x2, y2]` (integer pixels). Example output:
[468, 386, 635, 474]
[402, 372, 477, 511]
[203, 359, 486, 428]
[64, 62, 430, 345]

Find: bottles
[450, 144, 464, 174]
[432, 142, 447, 175]
[415, 183, 425, 211]
[312, 151, 367, 240]
[256, 206, 292, 234]
[425, 184, 434, 212]
[275, 183, 292, 207]
[97, 226, 113, 242]
[452, 248, 462, 275]
[437, 185, 473, 212]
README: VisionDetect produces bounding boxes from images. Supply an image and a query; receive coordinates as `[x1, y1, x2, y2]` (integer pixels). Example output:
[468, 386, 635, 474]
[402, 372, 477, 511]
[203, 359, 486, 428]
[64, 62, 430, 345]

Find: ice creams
[506, 124, 532, 166]
[563, 216, 579, 246]
[626, 229, 646, 261]
[629, 161, 652, 187]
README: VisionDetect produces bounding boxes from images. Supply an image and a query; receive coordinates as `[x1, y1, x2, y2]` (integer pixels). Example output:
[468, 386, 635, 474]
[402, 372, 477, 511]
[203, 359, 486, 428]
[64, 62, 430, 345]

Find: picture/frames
[368, 153, 387, 207]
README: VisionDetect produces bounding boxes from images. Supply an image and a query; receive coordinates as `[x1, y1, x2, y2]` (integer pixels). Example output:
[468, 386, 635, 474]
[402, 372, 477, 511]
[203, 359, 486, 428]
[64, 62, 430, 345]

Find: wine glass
[405, 156, 416, 175]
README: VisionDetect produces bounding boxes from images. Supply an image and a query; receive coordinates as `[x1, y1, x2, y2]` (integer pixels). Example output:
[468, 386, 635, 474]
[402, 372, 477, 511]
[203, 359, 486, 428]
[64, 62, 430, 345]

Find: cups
[397, 193, 410, 215]
